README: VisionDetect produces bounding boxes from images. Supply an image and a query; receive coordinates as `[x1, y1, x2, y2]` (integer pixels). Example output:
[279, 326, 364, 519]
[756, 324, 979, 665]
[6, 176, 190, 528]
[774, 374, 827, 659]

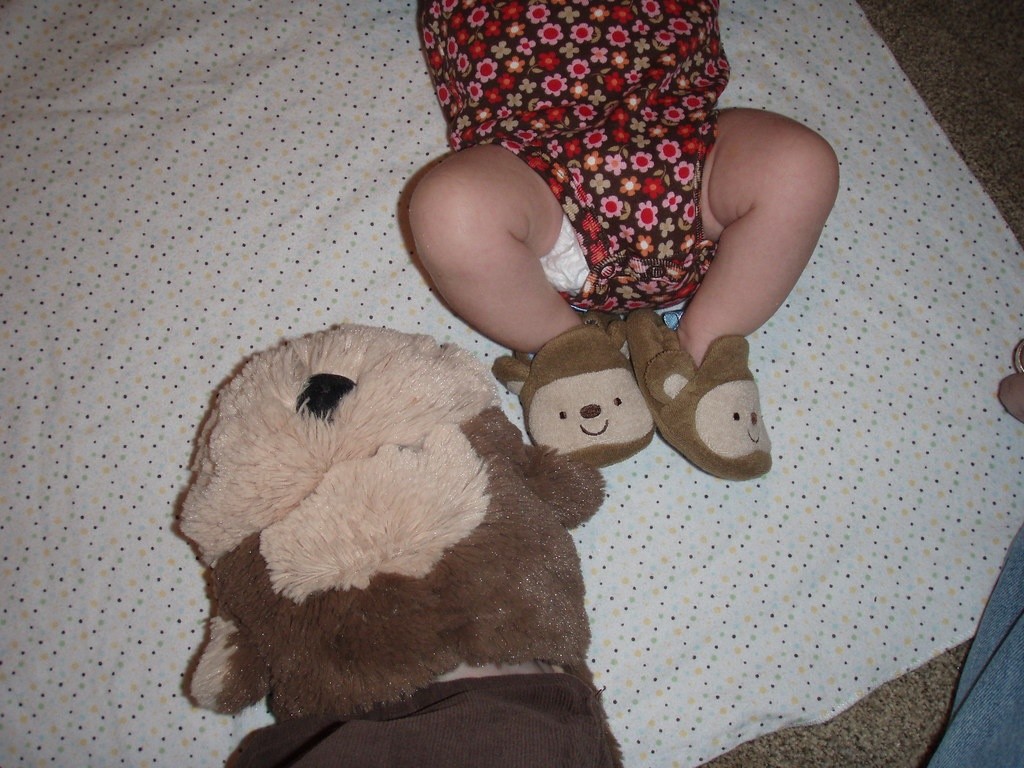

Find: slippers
[491, 309, 655, 469]
[628, 309, 772, 483]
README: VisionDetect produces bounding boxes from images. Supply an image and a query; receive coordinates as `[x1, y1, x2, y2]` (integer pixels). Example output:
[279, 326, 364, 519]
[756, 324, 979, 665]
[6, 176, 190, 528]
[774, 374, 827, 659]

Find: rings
[1015, 340, 1024, 372]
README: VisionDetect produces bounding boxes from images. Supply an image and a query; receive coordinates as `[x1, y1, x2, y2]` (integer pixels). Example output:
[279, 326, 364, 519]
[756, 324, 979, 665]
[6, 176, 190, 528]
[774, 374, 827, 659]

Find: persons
[180, 325, 623, 768]
[406, 0, 840, 482]
[926, 340, 1024, 768]
